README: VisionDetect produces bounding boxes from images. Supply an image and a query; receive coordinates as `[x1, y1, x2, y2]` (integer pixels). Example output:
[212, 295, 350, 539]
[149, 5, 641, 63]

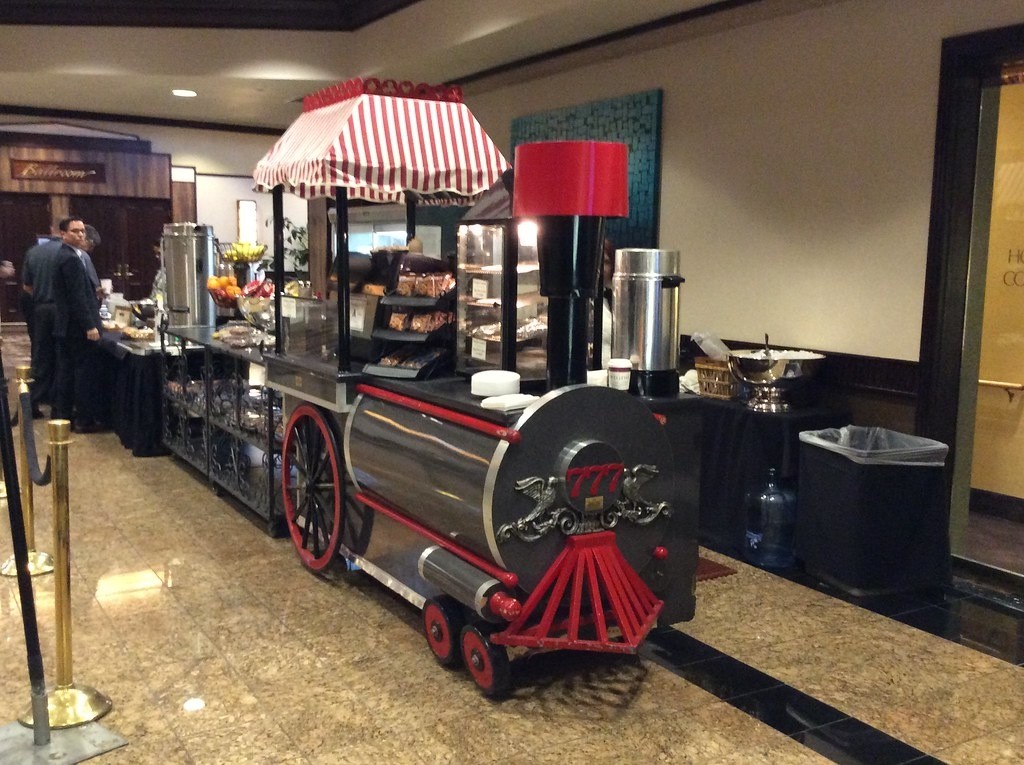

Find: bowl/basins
[726, 347, 826, 411]
[234, 295, 302, 331]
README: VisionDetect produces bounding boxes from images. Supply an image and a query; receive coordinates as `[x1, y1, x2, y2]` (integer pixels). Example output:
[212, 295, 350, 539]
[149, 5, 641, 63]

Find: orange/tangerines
[207, 275, 241, 296]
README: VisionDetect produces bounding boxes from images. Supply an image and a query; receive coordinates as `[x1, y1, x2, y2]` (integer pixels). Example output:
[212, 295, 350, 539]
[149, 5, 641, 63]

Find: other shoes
[75, 421, 108, 433]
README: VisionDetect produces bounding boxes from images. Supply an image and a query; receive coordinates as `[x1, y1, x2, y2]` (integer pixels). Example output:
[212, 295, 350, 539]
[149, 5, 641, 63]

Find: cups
[607, 357, 633, 393]
[700, 334, 731, 362]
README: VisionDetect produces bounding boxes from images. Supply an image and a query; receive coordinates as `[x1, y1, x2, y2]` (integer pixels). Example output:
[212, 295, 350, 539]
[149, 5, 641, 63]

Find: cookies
[389, 274, 456, 332]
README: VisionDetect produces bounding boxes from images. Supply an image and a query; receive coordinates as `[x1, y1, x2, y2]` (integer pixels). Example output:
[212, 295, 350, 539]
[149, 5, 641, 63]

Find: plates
[469, 369, 521, 399]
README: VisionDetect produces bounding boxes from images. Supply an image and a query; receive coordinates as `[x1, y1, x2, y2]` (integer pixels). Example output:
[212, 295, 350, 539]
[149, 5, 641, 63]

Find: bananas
[223, 240, 264, 261]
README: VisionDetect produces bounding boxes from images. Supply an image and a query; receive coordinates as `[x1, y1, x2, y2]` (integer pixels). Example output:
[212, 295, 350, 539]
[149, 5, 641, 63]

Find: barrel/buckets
[163, 222, 220, 334]
[612, 247, 684, 398]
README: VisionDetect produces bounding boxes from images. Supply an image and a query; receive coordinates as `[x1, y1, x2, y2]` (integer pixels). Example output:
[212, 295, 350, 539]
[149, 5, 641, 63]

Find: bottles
[743, 465, 799, 567]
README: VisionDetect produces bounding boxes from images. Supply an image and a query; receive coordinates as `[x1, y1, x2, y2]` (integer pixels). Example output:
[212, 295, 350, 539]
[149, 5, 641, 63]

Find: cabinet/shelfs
[159, 326, 297, 538]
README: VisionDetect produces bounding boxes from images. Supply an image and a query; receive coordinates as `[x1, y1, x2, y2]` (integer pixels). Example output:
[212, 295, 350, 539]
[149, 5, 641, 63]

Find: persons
[140, 237, 168, 298]
[21, 217, 107, 433]
[0, 260, 15, 278]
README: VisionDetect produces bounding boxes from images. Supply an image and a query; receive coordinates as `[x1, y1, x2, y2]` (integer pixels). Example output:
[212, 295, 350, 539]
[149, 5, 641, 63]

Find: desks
[102, 331, 250, 458]
[703, 398, 853, 528]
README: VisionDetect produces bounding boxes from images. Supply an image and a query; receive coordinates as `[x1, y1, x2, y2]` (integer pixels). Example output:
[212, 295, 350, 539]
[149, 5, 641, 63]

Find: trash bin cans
[798, 424, 949, 597]
